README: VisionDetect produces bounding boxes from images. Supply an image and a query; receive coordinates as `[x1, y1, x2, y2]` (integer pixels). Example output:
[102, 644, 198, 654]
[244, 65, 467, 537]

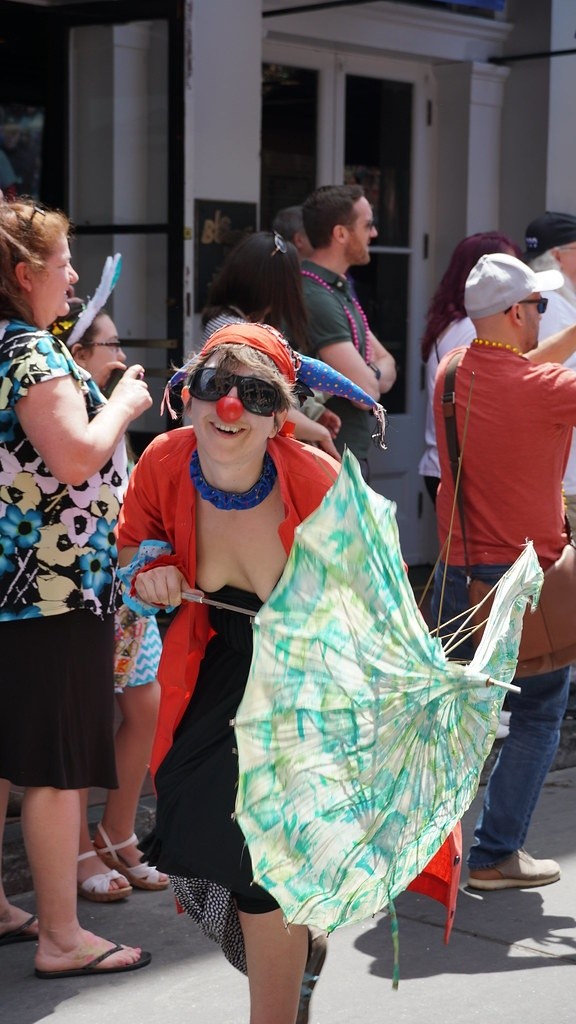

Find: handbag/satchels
[465, 540, 576, 681]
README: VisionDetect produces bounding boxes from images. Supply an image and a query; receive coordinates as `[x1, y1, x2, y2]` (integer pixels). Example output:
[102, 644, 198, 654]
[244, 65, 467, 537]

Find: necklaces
[185, 447, 277, 510]
[473, 338, 567, 512]
[298, 269, 371, 365]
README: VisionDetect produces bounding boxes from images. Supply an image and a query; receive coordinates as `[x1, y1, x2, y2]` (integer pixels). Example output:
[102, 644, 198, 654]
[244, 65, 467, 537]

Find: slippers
[33, 938, 152, 979]
[0, 914, 45, 945]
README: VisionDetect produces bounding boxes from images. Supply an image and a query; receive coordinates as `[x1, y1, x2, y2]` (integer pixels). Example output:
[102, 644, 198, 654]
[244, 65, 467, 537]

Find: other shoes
[466, 849, 561, 891]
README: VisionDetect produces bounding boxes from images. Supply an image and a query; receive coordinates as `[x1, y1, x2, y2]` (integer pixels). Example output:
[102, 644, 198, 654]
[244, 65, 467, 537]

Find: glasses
[269, 227, 288, 257]
[25, 203, 48, 231]
[187, 366, 284, 418]
[347, 221, 374, 231]
[504, 297, 549, 315]
[75, 338, 123, 354]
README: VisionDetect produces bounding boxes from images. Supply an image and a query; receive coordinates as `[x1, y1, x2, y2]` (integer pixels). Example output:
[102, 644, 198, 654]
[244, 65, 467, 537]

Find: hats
[522, 210, 576, 263]
[462, 252, 565, 320]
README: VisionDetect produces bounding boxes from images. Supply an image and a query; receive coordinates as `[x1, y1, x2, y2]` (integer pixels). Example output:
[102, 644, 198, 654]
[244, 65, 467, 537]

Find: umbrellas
[161, 443, 543, 988]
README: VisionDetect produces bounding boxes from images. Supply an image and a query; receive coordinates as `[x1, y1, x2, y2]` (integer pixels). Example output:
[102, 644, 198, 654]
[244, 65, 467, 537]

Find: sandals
[91, 820, 170, 891]
[75, 850, 133, 902]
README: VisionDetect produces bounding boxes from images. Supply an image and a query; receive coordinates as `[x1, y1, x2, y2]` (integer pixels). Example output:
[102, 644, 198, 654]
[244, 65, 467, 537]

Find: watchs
[368, 362, 381, 380]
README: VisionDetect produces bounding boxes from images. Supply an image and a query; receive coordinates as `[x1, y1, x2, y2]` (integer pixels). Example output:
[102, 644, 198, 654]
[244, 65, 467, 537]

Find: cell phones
[104, 368, 145, 398]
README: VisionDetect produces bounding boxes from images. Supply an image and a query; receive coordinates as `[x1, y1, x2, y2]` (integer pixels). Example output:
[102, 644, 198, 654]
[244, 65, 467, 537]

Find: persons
[199, 233, 339, 456]
[0, 199, 152, 979]
[51, 288, 162, 903]
[419, 210, 576, 888]
[272, 183, 396, 480]
[117, 326, 464, 1024]
